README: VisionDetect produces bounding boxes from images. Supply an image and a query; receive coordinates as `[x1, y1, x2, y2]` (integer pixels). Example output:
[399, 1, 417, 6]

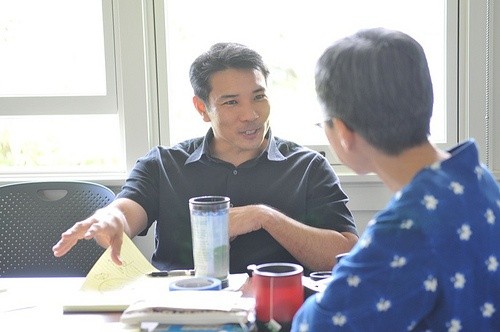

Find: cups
[189, 196, 230, 288]
[253, 263, 304, 332]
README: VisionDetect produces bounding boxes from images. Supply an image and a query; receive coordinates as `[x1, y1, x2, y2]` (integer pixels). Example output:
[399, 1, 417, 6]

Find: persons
[51, 42, 360, 275]
[292, 28, 499, 331]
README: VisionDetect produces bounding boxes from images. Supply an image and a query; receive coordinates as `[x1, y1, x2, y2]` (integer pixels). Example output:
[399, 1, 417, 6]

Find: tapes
[169, 276, 222, 292]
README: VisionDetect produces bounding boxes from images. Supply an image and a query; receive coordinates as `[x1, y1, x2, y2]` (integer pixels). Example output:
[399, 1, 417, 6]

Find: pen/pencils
[146, 270, 195, 278]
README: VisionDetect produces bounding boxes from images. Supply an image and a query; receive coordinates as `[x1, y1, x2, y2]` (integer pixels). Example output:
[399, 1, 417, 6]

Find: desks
[0, 278, 122, 332]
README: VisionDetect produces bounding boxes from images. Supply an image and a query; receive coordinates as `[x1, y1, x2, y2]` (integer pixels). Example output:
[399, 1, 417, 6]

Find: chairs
[0, 182, 116, 278]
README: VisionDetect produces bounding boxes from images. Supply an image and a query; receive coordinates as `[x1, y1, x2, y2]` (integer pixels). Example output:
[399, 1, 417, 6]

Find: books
[121, 292, 255, 327]
[62, 233, 160, 313]
